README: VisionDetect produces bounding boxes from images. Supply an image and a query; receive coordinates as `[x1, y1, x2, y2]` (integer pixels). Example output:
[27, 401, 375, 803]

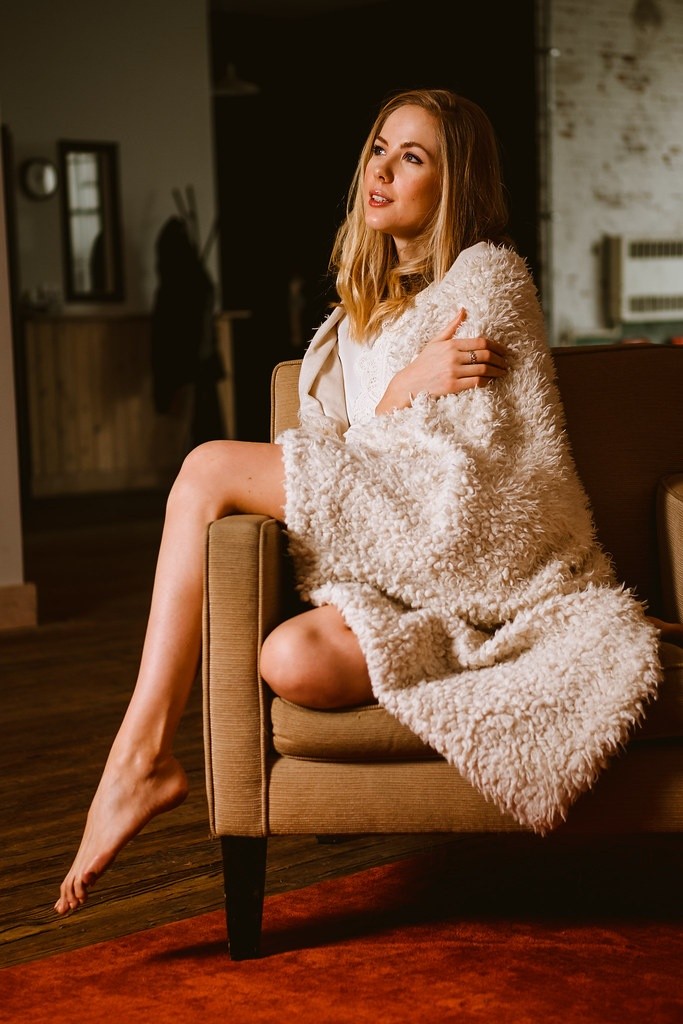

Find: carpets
[0, 860, 683, 1024]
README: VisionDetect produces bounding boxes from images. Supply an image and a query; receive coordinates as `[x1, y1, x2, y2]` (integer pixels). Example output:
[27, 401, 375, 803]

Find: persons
[55, 87, 661, 915]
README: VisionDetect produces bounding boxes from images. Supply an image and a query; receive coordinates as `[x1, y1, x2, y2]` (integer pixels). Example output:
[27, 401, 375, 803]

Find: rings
[470, 350, 477, 363]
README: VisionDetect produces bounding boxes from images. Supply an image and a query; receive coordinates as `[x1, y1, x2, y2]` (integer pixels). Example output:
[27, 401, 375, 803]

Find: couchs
[200, 342, 682, 962]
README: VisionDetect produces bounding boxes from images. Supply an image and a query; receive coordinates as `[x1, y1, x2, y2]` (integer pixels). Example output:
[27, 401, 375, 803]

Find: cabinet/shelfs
[17, 308, 251, 498]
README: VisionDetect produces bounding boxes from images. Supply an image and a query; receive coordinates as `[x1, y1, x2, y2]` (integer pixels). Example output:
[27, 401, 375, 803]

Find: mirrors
[56, 137, 122, 304]
[20, 155, 59, 201]
